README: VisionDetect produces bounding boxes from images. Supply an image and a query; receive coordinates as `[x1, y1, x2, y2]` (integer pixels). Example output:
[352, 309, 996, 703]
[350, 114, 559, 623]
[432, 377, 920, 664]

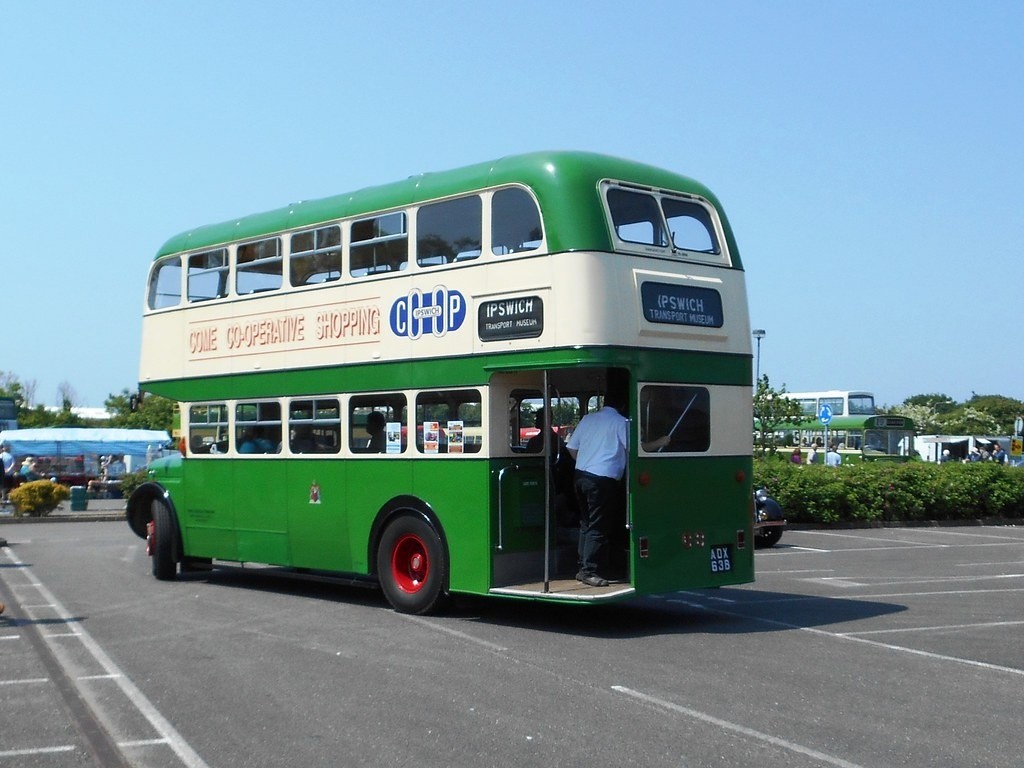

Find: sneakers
[576, 573, 582, 580]
[582, 575, 608, 587]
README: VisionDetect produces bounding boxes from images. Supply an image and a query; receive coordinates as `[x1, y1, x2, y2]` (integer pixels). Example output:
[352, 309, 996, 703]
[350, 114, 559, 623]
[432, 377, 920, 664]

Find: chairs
[189, 245, 538, 303]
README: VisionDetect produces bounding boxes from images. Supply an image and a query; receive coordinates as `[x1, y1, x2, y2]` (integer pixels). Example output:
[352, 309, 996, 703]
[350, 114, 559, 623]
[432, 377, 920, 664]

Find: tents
[0, 428, 172, 504]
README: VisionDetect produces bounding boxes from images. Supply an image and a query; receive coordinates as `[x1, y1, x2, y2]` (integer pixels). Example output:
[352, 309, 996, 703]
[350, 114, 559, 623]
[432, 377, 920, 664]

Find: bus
[753, 389, 919, 465]
[126, 148, 758, 622]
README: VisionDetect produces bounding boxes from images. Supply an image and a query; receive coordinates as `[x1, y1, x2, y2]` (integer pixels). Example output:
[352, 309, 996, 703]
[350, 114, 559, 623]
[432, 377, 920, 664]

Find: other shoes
[608, 573, 629, 583]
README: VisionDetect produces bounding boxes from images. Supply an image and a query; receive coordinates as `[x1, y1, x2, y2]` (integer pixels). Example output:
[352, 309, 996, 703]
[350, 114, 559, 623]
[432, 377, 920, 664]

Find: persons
[108, 453, 126, 475]
[526, 408, 564, 453]
[792, 432, 800, 446]
[20, 457, 45, 482]
[813, 437, 822, 451]
[941, 449, 951, 462]
[927, 446, 930, 461]
[0, 447, 16, 504]
[966, 444, 1008, 466]
[366, 411, 387, 454]
[807, 443, 818, 464]
[566, 390, 671, 586]
[792, 448, 802, 465]
[824, 445, 841, 467]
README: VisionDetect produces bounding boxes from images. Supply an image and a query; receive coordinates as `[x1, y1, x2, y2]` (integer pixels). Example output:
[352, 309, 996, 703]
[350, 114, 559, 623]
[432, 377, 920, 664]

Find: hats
[530, 408, 553, 420]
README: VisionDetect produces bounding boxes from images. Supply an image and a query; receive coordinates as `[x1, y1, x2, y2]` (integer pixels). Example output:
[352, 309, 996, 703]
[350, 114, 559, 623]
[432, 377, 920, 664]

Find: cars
[753, 483, 788, 550]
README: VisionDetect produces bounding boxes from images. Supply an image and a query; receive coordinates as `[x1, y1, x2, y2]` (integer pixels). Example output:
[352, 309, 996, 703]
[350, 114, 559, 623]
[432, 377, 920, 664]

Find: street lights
[933, 400, 952, 414]
[753, 329, 766, 392]
